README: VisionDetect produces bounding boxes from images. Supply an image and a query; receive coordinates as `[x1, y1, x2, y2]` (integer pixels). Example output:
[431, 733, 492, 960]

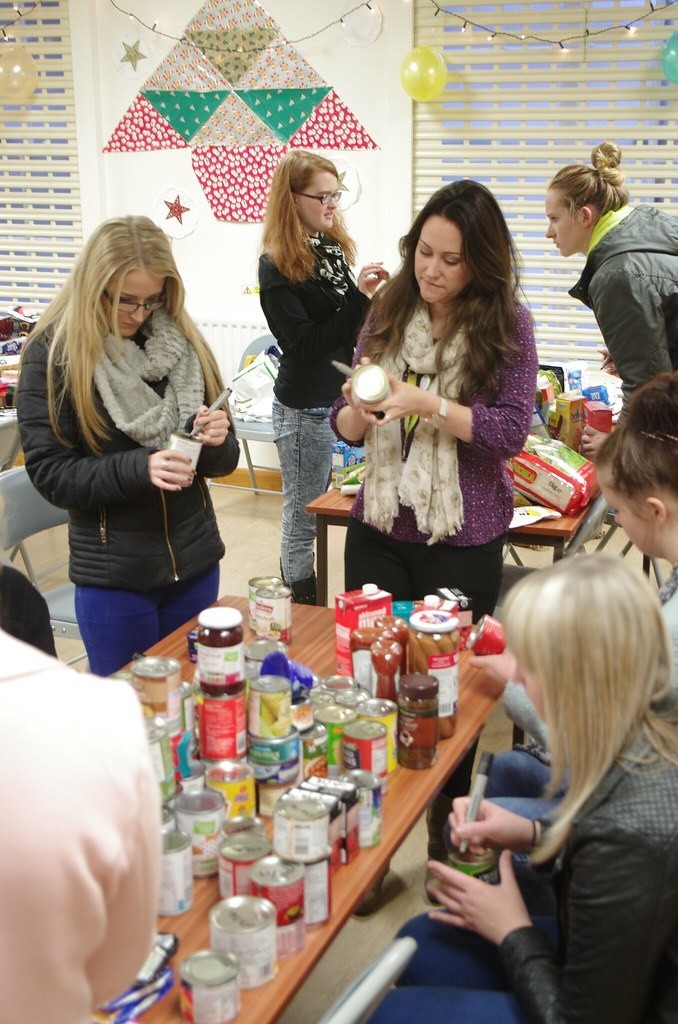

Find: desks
[99, 591, 501, 1022]
[309, 438, 611, 611]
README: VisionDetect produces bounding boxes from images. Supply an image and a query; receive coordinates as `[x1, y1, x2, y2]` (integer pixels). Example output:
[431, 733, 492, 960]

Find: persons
[544, 142, 677, 463]
[0, 631, 163, 1024]
[458, 366, 678, 867]
[255, 146, 389, 607]
[330, 179, 538, 916]
[12, 214, 239, 689]
[361, 546, 678, 1024]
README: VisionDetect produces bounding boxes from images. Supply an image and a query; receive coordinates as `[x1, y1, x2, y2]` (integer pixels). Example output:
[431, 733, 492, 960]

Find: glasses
[102, 287, 168, 314]
[292, 191, 342, 205]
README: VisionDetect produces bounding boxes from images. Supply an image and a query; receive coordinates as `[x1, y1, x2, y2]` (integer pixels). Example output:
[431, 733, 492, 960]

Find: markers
[457, 750, 495, 857]
[328, 357, 355, 379]
[189, 387, 232, 440]
[600, 357, 612, 372]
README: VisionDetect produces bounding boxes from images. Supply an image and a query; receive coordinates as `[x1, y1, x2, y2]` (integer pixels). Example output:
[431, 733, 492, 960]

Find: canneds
[253, 586, 295, 645]
[445, 840, 499, 889]
[247, 576, 287, 633]
[349, 365, 394, 407]
[163, 430, 202, 489]
[120, 646, 399, 1023]
[467, 614, 510, 657]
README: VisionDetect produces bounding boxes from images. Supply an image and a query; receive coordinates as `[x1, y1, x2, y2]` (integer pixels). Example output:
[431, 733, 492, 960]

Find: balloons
[659, 30, 678, 88]
[401, 43, 447, 103]
[0, 45, 39, 97]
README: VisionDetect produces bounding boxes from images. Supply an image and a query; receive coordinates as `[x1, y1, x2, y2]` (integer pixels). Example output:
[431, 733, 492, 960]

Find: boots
[282, 573, 317, 607]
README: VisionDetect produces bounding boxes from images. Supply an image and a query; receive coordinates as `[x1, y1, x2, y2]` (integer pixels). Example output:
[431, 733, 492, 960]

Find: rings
[160, 460, 170, 469]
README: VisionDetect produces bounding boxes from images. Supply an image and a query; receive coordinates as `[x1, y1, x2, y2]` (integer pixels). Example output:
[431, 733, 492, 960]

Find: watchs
[423, 396, 448, 429]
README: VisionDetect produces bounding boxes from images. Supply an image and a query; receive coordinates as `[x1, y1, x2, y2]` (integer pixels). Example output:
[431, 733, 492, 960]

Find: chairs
[2, 468, 98, 637]
[205, 333, 289, 498]
